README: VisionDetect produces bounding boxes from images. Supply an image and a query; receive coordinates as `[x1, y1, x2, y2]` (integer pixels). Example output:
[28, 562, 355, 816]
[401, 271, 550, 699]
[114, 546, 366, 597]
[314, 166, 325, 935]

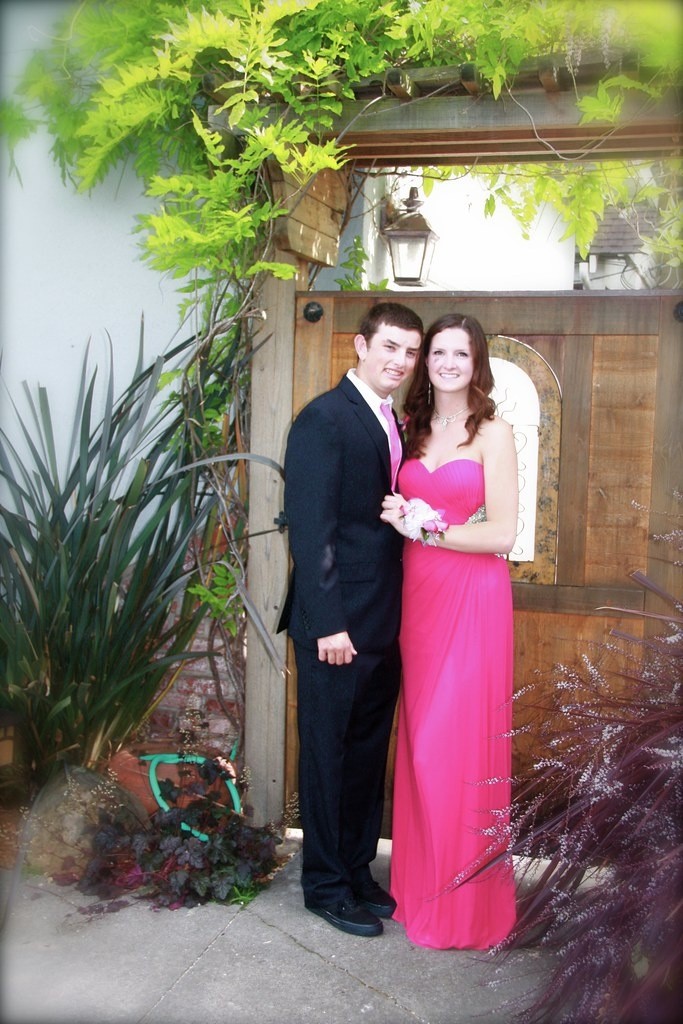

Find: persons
[276, 303, 423, 937]
[381, 312, 518, 948]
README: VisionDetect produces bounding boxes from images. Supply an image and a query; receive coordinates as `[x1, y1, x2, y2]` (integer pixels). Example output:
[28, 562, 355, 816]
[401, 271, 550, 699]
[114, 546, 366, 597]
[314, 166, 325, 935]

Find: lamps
[380, 187, 439, 287]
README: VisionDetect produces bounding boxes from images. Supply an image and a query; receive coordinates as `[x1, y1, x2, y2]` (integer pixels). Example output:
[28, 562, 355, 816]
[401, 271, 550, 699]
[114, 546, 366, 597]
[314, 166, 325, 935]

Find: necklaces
[432, 407, 469, 426]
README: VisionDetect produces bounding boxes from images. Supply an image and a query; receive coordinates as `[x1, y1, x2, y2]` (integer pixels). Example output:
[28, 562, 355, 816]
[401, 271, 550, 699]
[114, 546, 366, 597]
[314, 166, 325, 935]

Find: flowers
[398, 498, 451, 547]
[398, 415, 410, 431]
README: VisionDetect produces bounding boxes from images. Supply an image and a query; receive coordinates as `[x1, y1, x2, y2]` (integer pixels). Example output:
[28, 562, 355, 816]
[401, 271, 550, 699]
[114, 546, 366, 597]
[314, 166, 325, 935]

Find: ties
[379, 400, 404, 493]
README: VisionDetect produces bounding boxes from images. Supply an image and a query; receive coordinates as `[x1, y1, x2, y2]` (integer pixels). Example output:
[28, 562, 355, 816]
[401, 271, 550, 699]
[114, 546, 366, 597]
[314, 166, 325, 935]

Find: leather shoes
[348, 883, 397, 922]
[303, 890, 385, 939]
